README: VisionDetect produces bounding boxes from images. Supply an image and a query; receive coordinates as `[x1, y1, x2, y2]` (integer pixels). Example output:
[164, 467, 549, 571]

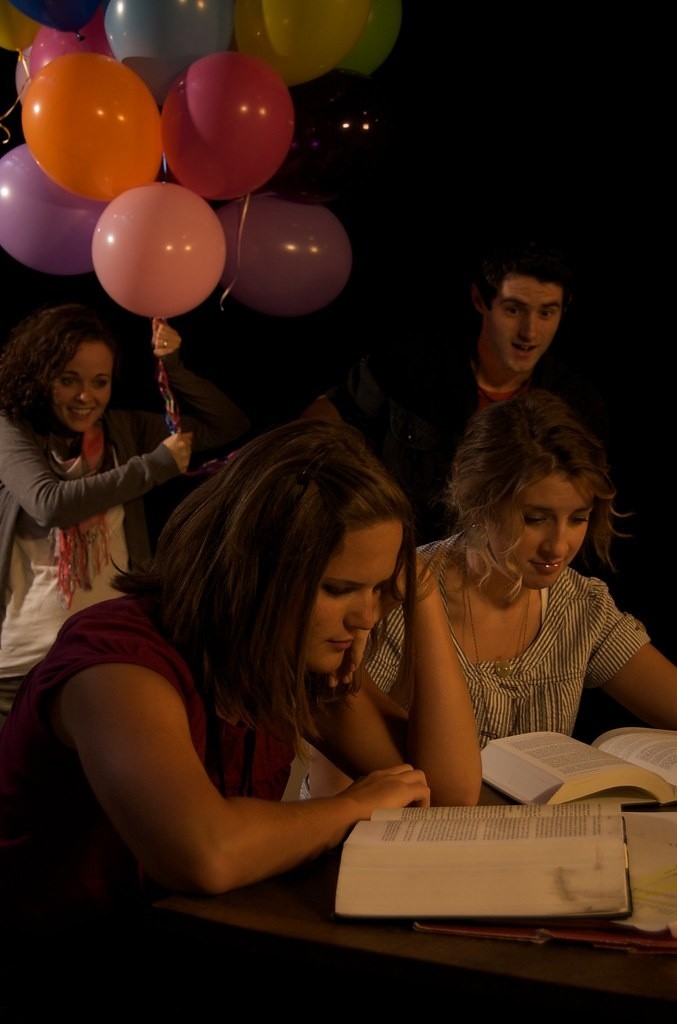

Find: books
[480, 720, 677, 805]
[334, 800, 633, 921]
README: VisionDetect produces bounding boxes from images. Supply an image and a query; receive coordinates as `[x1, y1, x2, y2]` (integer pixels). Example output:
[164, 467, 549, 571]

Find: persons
[364, 239, 573, 548]
[359, 391, 677, 758]
[0, 304, 251, 725]
[0, 418, 482, 944]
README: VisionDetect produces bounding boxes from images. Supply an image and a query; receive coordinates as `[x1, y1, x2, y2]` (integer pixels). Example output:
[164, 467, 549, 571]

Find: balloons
[215, 192, 353, 315]
[234, 0, 372, 88]
[336, 0, 403, 74]
[162, 51, 296, 200]
[22, 52, 163, 202]
[0, 0, 232, 103]
[91, 183, 227, 319]
[0, 143, 110, 275]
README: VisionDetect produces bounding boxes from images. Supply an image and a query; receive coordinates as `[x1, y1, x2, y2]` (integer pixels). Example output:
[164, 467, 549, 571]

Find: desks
[144, 782, 677, 1003]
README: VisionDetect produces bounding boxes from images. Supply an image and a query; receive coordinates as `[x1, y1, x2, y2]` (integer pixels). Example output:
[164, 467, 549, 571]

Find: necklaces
[464, 582, 530, 677]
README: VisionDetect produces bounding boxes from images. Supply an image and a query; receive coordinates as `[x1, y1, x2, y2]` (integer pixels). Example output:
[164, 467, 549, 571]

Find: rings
[163, 341, 168, 347]
